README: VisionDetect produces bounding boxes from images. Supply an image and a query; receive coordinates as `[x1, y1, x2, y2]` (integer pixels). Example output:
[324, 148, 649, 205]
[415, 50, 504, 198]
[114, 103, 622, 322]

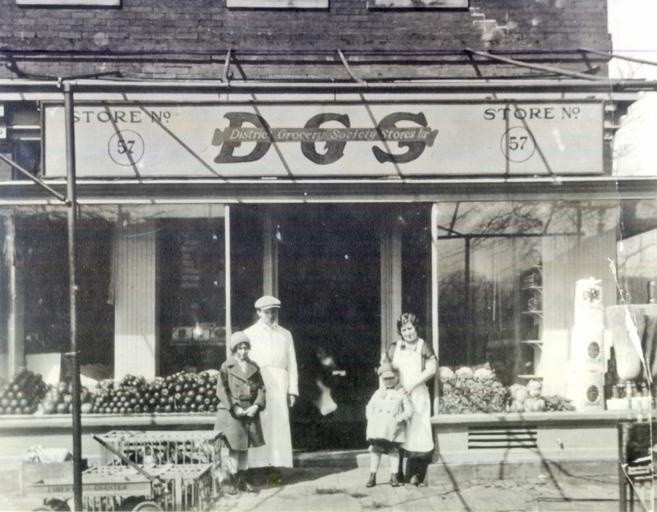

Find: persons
[365, 312, 438, 488]
[213, 295, 299, 494]
[304, 341, 348, 454]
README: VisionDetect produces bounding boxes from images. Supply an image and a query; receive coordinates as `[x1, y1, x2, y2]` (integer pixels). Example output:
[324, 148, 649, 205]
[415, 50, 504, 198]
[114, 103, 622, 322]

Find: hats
[377, 362, 395, 379]
[254, 296, 282, 310]
[229, 331, 251, 352]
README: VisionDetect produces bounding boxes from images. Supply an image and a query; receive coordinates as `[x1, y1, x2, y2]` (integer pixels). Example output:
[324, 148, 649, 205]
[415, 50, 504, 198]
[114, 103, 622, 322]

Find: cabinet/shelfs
[502, 199, 620, 411]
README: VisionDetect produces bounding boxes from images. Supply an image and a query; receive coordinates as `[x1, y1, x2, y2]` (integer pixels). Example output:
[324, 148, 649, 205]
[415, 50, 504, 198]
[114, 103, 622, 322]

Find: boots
[366, 472, 376, 487]
[390, 474, 399, 486]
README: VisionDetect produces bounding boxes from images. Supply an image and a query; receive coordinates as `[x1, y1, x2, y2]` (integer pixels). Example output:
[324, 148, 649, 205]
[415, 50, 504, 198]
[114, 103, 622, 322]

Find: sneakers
[409, 475, 420, 485]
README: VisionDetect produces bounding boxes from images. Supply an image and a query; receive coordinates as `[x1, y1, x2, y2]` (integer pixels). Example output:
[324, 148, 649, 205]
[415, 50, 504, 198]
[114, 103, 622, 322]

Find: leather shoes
[220, 468, 289, 495]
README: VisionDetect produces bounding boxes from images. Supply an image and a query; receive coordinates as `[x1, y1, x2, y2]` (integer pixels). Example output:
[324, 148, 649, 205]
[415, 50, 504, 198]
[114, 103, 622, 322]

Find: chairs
[18, 429, 228, 512]
